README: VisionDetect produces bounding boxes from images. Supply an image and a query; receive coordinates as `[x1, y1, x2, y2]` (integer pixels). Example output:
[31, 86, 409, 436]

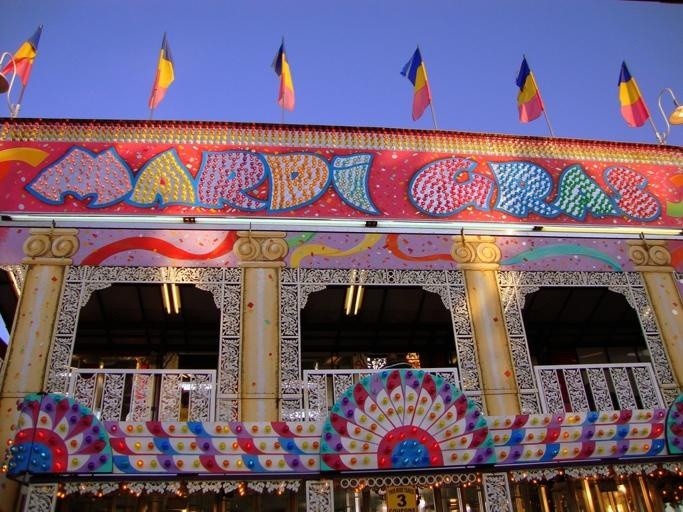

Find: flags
[398, 50, 430, 120]
[515, 57, 544, 123]
[616, 59, 650, 126]
[272, 44, 295, 113]
[1, 28, 45, 90]
[153, 37, 175, 111]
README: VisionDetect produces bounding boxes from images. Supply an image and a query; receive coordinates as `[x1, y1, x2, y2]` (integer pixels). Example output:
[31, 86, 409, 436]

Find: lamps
[160, 267, 181, 315]
[8, 267, 24, 296]
[344, 270, 367, 316]
